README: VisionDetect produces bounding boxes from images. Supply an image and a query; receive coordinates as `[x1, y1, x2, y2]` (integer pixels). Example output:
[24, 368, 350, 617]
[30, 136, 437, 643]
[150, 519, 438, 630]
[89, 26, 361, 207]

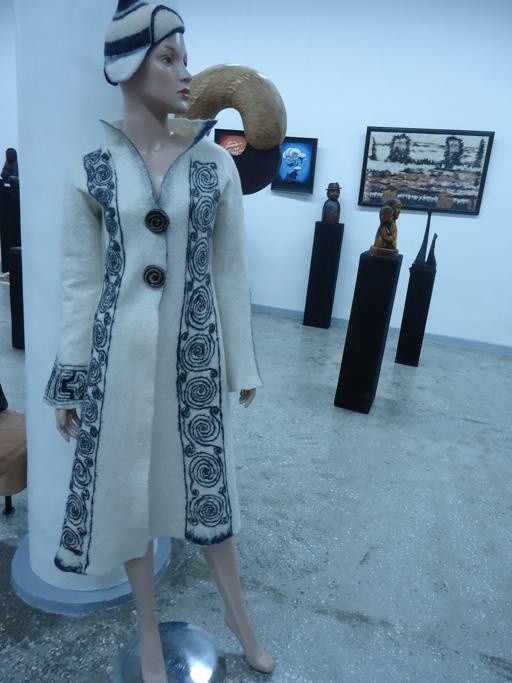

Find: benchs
[0, 408, 27, 516]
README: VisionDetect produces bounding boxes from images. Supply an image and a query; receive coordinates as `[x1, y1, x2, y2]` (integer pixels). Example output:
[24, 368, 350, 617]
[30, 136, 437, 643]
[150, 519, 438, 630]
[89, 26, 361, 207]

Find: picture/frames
[270, 137, 318, 194]
[214, 128, 248, 156]
[357, 126, 495, 215]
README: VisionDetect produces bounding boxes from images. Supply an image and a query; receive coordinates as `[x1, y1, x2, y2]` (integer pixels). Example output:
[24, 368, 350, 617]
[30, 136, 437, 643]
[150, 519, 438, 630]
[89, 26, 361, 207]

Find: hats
[103, 0, 185, 86]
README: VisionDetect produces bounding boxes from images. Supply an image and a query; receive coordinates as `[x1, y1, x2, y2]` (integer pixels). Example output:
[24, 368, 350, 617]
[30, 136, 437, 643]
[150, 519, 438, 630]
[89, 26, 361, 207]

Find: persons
[42, 0, 276, 682]
[374, 206, 395, 248]
[321, 181, 343, 223]
[385, 197, 401, 247]
